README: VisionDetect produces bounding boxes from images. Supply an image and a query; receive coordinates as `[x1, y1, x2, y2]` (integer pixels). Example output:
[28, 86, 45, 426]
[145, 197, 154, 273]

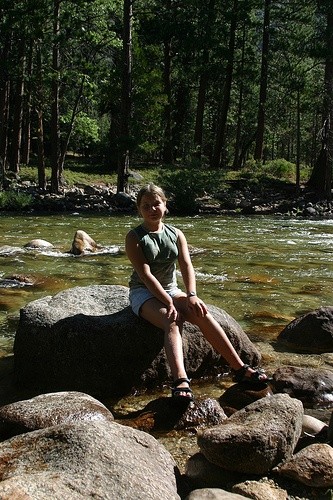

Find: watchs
[187, 291, 198, 296]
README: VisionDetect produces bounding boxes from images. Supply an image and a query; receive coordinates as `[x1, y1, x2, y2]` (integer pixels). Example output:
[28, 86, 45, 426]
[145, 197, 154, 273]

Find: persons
[124, 182, 270, 401]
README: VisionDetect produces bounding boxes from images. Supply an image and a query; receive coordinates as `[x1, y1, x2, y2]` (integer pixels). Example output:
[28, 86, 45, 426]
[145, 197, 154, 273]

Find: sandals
[171, 377, 194, 402]
[230, 364, 276, 385]
